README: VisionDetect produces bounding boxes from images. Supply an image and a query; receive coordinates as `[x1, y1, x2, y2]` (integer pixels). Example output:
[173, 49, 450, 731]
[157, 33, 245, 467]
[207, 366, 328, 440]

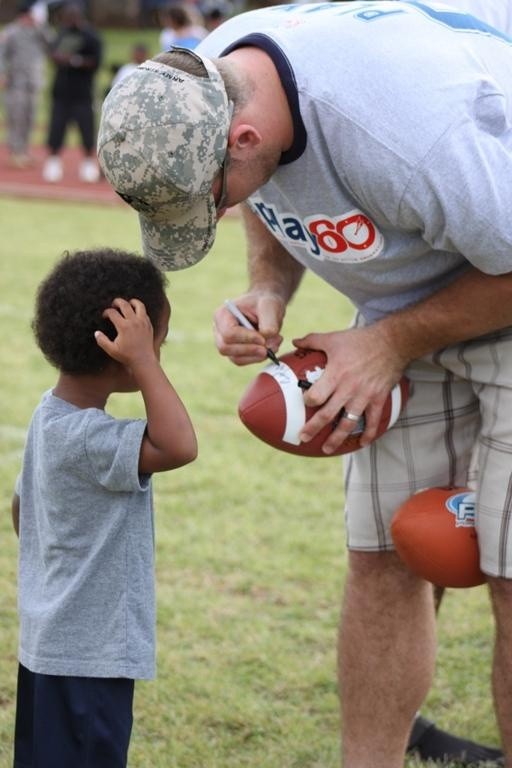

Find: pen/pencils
[225, 298, 280, 366]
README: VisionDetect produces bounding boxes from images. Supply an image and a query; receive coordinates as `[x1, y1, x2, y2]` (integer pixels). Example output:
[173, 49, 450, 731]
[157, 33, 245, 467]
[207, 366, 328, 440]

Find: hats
[96, 44, 230, 273]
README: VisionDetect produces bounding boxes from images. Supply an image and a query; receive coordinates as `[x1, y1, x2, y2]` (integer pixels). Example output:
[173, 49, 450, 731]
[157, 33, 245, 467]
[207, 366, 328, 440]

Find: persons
[1, 6, 43, 170]
[37, 1, 100, 185]
[11, 245, 198, 767]
[105, 1, 223, 95]
[101, 0, 512, 768]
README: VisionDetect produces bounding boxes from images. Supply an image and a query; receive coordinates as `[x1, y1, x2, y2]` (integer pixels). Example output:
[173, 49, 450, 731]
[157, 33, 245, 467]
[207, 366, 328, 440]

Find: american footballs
[237, 351, 411, 458]
[391, 486, 485, 589]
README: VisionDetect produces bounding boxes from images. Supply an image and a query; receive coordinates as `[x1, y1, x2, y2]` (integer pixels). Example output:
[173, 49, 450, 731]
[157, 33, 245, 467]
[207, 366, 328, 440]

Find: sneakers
[7, 153, 102, 185]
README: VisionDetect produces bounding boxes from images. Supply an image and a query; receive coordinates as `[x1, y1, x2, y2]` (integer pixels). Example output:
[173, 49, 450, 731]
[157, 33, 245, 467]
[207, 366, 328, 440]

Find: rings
[341, 412, 361, 423]
